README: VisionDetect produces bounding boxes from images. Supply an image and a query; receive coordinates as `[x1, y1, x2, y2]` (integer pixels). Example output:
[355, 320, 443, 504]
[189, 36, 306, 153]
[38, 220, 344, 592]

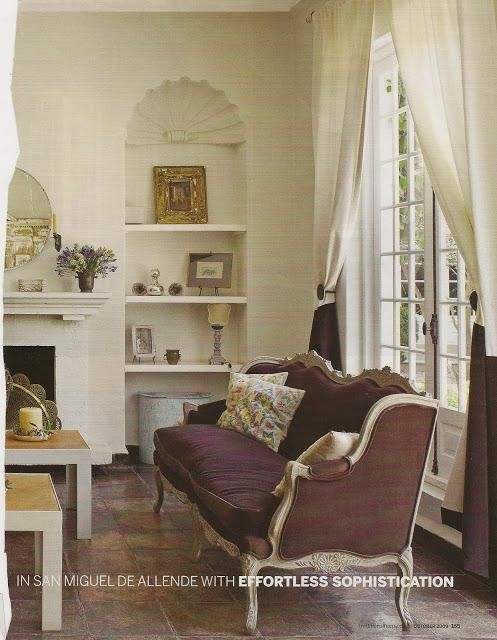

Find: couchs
[152, 350, 438, 636]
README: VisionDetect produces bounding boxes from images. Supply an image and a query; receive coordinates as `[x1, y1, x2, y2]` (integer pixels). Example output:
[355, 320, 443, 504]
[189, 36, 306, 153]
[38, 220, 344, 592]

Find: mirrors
[5, 165, 53, 272]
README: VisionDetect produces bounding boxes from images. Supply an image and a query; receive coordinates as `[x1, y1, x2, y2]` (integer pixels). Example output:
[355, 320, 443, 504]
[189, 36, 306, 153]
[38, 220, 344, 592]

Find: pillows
[216, 372, 287, 431]
[221, 380, 304, 453]
[272, 432, 359, 498]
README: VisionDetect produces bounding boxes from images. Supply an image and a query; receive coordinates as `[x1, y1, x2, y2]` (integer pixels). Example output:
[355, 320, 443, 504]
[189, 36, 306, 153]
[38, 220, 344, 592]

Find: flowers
[53, 243, 119, 279]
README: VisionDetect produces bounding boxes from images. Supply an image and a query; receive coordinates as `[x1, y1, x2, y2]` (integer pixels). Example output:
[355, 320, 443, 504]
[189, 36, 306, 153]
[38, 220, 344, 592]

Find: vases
[76, 271, 94, 292]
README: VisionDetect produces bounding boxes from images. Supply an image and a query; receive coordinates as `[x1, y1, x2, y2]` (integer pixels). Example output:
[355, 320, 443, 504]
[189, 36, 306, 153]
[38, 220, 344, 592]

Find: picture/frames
[132, 325, 157, 358]
[152, 166, 208, 223]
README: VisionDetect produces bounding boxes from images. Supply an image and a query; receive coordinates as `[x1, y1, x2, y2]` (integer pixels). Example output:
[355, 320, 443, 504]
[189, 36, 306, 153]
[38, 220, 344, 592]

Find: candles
[19, 407, 42, 435]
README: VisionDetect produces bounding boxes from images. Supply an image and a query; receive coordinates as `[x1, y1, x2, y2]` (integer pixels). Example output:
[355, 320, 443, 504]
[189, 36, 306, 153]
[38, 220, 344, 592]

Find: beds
[5, 473, 65, 631]
